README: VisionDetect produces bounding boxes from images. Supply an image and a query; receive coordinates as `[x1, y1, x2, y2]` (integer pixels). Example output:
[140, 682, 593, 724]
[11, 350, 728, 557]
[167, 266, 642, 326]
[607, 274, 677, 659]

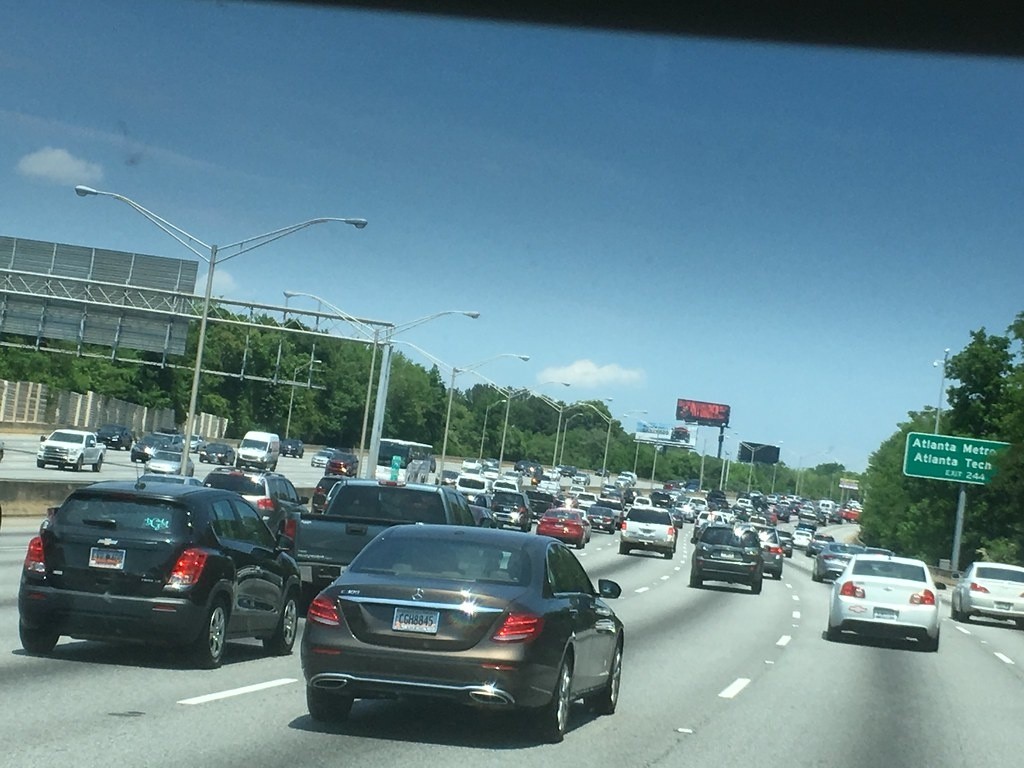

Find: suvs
[17, 480, 302, 670]
[309, 473, 360, 514]
[201, 466, 309, 546]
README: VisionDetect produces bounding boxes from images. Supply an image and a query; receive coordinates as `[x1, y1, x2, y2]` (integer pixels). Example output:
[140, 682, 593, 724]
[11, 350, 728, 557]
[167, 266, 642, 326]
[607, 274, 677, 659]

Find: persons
[680, 405, 691, 417]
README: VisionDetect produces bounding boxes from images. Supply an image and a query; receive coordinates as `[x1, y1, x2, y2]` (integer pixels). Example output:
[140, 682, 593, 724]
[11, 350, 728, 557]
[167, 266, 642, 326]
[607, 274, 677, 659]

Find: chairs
[357, 488, 381, 515]
[505, 549, 532, 586]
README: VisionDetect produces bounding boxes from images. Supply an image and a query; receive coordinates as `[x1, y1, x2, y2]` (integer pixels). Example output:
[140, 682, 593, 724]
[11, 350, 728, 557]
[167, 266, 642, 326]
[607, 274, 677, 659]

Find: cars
[199, 442, 236, 465]
[181, 435, 207, 454]
[136, 473, 202, 487]
[131, 436, 176, 464]
[95, 424, 133, 451]
[950, 561, 1024, 631]
[279, 437, 868, 595]
[142, 451, 194, 477]
[151, 432, 184, 453]
[826, 552, 947, 653]
[302, 521, 627, 743]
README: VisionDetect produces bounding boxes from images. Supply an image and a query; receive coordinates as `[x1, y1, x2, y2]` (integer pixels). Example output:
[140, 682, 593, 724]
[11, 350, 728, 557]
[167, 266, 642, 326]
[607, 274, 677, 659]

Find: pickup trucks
[36, 428, 107, 472]
[293, 480, 477, 595]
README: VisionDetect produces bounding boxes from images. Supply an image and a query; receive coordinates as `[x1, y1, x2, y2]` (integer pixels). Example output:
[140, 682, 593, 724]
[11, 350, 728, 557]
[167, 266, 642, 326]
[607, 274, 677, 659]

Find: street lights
[72, 184, 367, 441]
[933, 347, 951, 433]
[282, 290, 480, 475]
[726, 435, 784, 492]
[520, 383, 613, 470]
[386, 338, 530, 482]
[453, 366, 571, 474]
[572, 400, 648, 477]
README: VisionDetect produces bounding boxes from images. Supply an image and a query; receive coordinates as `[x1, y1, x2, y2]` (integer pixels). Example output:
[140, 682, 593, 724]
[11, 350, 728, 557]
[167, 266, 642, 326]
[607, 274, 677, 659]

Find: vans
[235, 430, 279, 471]
